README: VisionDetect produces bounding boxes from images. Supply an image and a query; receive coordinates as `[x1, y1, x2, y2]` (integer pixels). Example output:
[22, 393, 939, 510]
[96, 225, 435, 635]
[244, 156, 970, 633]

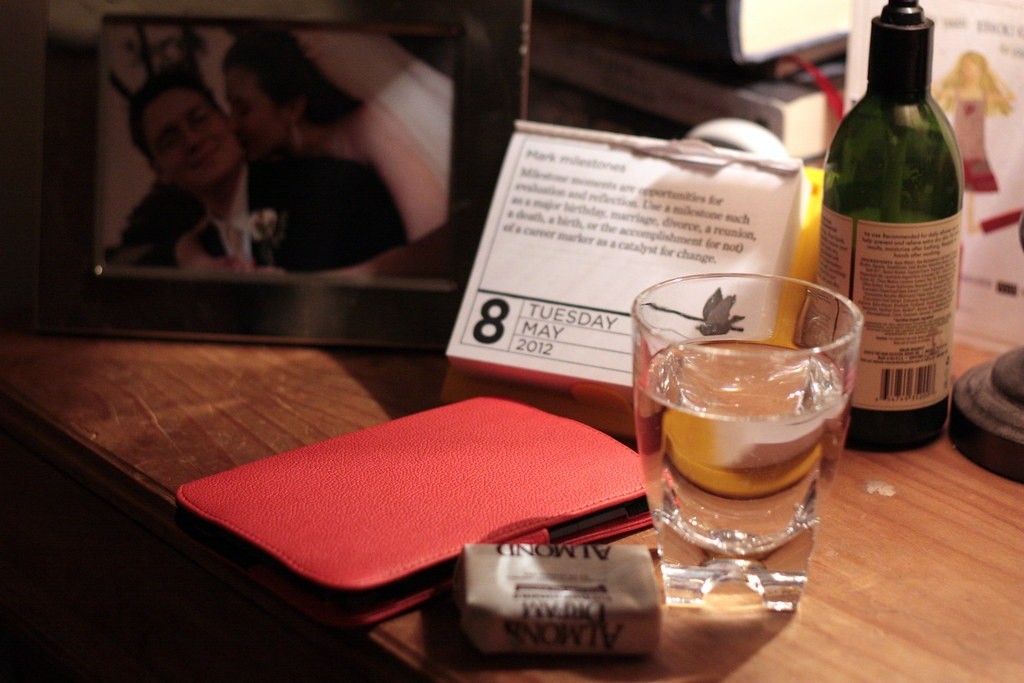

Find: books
[175, 394, 675, 628]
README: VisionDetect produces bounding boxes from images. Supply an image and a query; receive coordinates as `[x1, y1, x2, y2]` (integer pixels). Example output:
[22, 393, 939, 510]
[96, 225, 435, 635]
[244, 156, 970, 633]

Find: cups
[633, 273, 865, 612]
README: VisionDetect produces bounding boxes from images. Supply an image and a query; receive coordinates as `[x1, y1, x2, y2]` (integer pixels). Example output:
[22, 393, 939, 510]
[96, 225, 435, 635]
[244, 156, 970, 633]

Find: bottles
[815, 0, 964, 452]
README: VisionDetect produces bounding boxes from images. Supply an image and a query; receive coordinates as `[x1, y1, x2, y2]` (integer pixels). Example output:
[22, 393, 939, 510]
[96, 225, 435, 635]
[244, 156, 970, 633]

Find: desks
[1, 341, 1024, 683]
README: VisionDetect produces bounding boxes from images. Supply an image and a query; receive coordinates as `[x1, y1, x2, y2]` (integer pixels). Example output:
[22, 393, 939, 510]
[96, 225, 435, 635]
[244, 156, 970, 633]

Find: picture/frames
[40, 1, 536, 349]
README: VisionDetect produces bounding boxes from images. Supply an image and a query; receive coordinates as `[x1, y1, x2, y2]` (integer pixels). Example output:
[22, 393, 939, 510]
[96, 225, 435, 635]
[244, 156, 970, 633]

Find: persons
[121, 35, 449, 262]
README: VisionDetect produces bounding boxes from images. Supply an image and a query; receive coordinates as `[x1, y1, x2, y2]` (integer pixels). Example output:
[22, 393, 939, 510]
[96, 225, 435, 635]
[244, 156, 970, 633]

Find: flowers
[240, 205, 286, 270]
